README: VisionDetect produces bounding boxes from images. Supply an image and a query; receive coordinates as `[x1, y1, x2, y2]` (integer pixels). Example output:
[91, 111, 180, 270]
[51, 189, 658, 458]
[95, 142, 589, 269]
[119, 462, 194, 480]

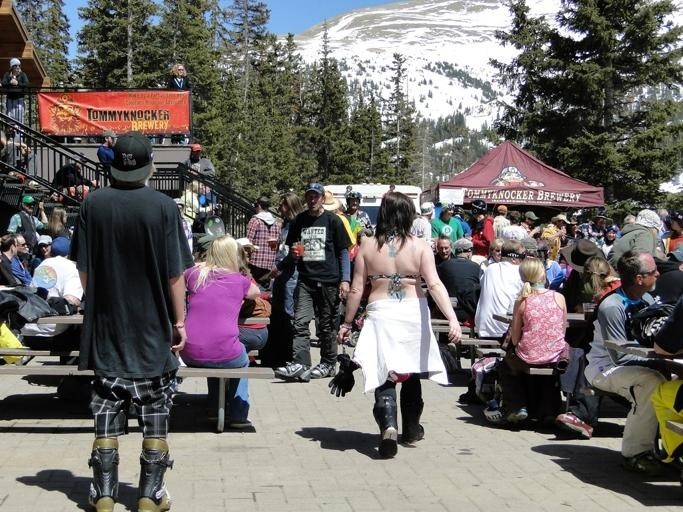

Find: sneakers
[207, 410, 231, 424]
[229, 418, 252, 429]
[310, 361, 337, 380]
[617, 447, 667, 478]
[274, 362, 311, 383]
[507, 405, 529, 426]
[555, 410, 597, 441]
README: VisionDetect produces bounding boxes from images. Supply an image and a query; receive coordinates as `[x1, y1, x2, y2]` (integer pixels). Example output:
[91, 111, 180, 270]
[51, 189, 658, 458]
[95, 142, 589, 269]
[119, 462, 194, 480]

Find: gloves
[328, 353, 360, 398]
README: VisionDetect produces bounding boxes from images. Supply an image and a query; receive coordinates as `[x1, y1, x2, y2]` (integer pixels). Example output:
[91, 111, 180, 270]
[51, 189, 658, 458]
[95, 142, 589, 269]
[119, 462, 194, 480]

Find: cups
[266, 239, 278, 251]
[583, 303, 597, 322]
[292, 242, 304, 257]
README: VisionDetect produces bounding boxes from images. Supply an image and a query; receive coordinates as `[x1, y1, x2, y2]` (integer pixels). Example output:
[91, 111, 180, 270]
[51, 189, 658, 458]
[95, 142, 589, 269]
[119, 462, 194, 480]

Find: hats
[558, 238, 606, 273]
[191, 142, 362, 212]
[22, 195, 70, 258]
[634, 208, 664, 234]
[103, 130, 118, 138]
[9, 57, 21, 66]
[173, 198, 186, 205]
[665, 245, 683, 263]
[420, 200, 620, 234]
[110, 129, 155, 182]
[670, 210, 683, 228]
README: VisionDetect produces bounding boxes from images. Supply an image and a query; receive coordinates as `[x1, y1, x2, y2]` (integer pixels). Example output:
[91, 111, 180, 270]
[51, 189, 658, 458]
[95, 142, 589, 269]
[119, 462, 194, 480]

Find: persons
[0, 118, 31, 182]
[97, 132, 116, 175]
[168, 63, 191, 144]
[68, 131, 195, 512]
[386, 185, 396, 193]
[337, 191, 462, 457]
[1, 180, 374, 383]
[407, 199, 683, 480]
[2, 58, 29, 141]
[48, 154, 100, 210]
[191, 144, 217, 206]
[182, 234, 261, 429]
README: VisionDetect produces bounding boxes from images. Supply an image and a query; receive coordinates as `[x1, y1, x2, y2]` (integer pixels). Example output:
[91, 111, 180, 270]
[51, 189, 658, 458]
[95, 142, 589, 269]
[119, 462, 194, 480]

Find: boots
[373, 395, 398, 457]
[400, 397, 425, 443]
[136, 437, 172, 512]
[86, 437, 119, 512]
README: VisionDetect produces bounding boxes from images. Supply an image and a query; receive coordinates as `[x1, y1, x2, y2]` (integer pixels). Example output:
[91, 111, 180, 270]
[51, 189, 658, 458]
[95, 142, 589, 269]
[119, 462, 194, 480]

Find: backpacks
[467, 350, 506, 407]
[630, 301, 676, 349]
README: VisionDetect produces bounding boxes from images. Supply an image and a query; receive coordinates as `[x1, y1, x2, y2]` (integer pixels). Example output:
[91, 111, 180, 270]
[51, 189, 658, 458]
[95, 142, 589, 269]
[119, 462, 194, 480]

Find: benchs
[0, 348, 80, 365]
[0, 365, 276, 433]
[430, 311, 683, 436]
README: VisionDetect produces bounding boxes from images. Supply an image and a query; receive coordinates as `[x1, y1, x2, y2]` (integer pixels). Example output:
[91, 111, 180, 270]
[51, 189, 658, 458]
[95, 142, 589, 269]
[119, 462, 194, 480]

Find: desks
[38, 312, 271, 431]
